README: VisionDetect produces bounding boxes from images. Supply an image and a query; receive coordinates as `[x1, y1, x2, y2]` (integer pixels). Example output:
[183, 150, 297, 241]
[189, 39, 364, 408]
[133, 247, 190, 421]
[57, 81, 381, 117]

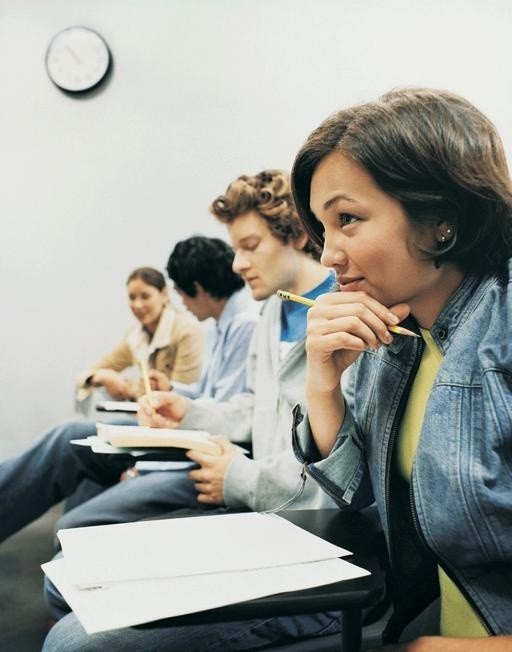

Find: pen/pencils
[137, 362, 156, 421]
[275, 288, 423, 337]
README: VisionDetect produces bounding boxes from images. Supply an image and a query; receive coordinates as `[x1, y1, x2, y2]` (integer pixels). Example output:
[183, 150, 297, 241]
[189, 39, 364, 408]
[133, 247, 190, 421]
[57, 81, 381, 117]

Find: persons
[37, 165, 388, 652]
[75, 266, 204, 408]
[287, 87, 511, 650]
[1, 235, 261, 545]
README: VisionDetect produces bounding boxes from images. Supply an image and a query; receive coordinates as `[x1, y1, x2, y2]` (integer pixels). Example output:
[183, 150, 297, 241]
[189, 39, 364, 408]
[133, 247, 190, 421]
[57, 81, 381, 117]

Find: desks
[42, 398, 395, 650]
